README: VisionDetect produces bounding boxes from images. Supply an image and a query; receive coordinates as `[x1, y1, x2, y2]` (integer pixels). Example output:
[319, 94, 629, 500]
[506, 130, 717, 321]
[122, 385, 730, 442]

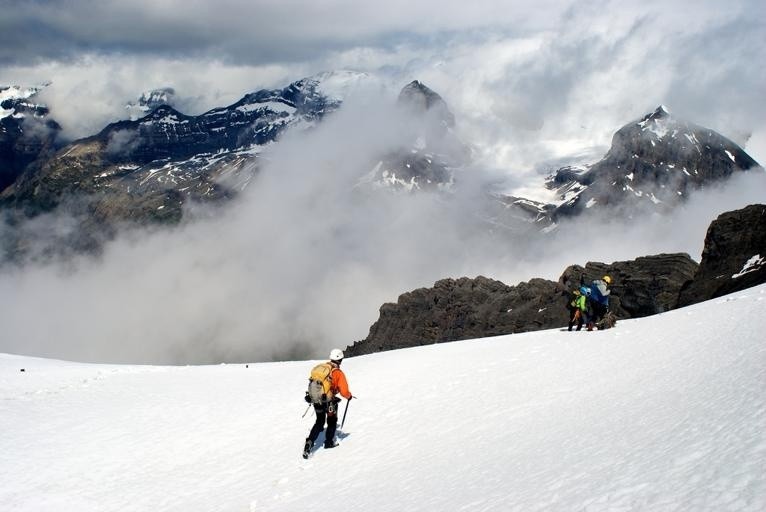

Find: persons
[588, 275, 611, 331]
[303, 348, 352, 459]
[568, 287, 591, 331]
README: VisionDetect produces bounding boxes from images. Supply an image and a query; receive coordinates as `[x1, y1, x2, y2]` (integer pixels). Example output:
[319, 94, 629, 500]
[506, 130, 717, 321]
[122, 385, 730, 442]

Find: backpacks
[308, 363, 339, 414]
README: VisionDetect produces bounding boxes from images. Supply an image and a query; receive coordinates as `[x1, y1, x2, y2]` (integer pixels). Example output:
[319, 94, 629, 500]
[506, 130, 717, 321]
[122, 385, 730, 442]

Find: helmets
[329, 348, 344, 361]
[580, 287, 587, 295]
[603, 276, 611, 284]
[586, 288, 592, 295]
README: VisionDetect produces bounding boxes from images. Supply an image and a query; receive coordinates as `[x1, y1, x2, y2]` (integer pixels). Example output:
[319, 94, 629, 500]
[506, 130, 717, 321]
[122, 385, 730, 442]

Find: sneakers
[303, 440, 312, 459]
[323, 440, 339, 449]
